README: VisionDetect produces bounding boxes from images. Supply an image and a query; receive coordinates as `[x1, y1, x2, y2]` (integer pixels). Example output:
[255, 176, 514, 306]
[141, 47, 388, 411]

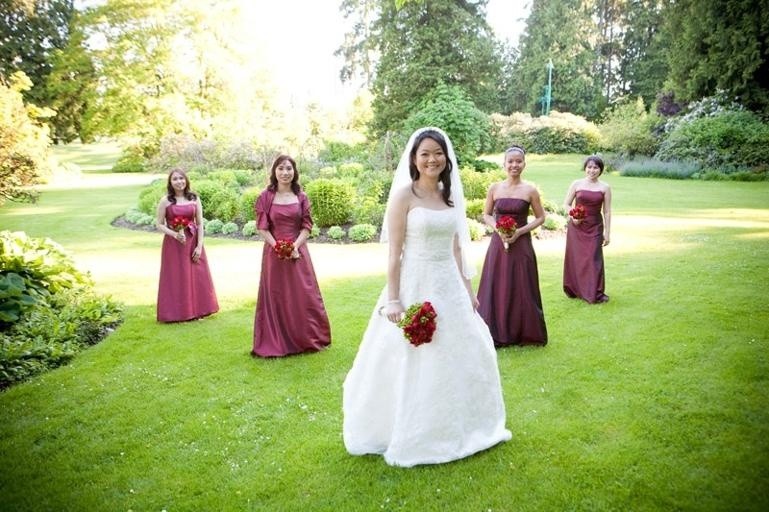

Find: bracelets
[386, 299, 399, 305]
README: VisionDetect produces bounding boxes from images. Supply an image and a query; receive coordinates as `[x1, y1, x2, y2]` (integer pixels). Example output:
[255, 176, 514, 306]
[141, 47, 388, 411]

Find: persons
[338, 124, 514, 471]
[561, 154, 612, 306]
[473, 143, 549, 348]
[152, 166, 220, 325]
[249, 152, 332, 359]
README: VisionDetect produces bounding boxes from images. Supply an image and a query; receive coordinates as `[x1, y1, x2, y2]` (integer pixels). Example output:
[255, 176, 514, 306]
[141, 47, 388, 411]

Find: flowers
[169, 215, 191, 243]
[377, 301, 438, 347]
[273, 239, 302, 262]
[569, 204, 588, 228]
[496, 216, 517, 253]
